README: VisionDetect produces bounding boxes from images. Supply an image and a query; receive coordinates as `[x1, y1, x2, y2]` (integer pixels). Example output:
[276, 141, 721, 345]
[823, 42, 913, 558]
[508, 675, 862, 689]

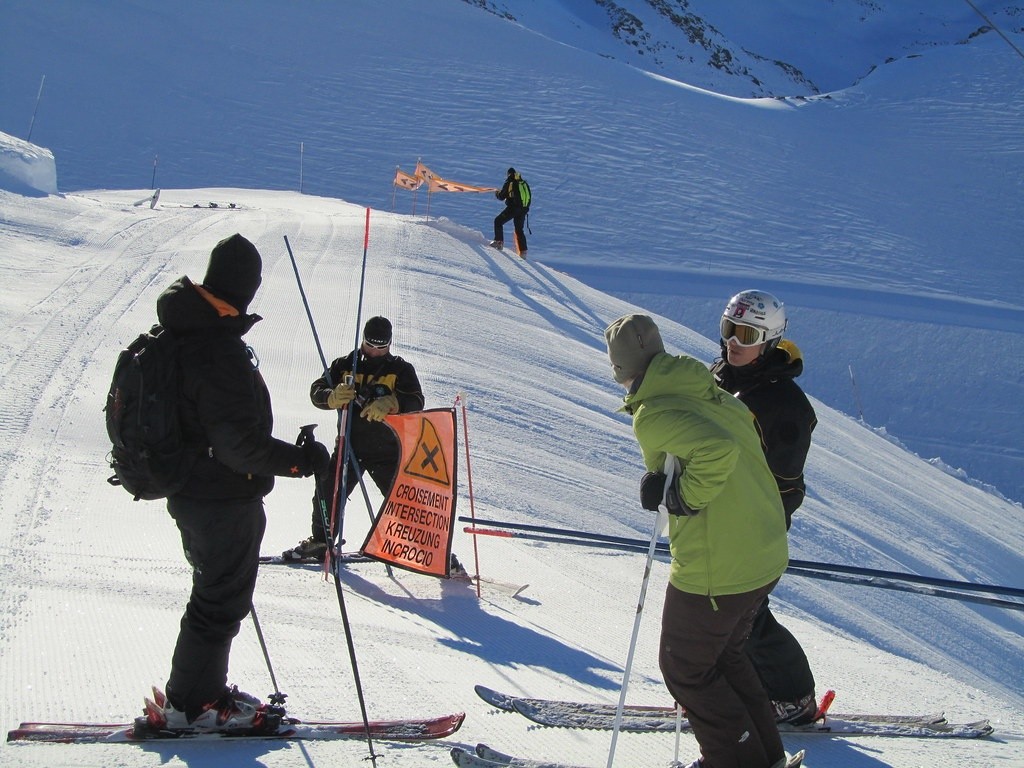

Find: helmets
[720, 289, 785, 350]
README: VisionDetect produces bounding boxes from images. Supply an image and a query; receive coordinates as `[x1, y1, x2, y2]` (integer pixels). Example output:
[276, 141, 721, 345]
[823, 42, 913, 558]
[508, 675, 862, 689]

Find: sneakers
[282, 542, 329, 561]
[770, 689, 818, 729]
[162, 683, 286, 732]
[518, 250, 528, 260]
[684, 756, 786, 768]
[451, 553, 465, 576]
[489, 241, 504, 251]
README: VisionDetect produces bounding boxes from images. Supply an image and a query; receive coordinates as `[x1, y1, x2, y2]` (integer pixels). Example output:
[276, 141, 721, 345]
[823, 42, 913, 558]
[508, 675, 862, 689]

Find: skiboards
[6, 710, 468, 745]
[160, 200, 261, 211]
[258, 546, 474, 584]
[474, 683, 993, 742]
[451, 743, 807, 768]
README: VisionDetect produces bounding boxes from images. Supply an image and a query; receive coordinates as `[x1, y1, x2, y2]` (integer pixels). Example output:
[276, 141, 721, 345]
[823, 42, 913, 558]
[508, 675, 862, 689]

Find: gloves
[641, 472, 700, 522]
[360, 395, 397, 423]
[328, 383, 355, 409]
[302, 442, 330, 477]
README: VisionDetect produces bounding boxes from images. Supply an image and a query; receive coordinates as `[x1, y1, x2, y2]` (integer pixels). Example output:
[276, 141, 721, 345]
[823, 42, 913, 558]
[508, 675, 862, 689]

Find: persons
[159, 233, 329, 733]
[282, 316, 460, 575]
[704, 290, 817, 728]
[489, 167, 531, 260]
[604, 313, 789, 768]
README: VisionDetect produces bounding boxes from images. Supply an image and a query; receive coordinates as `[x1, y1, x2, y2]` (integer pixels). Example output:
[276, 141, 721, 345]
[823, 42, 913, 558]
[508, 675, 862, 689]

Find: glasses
[364, 339, 392, 350]
[720, 317, 784, 347]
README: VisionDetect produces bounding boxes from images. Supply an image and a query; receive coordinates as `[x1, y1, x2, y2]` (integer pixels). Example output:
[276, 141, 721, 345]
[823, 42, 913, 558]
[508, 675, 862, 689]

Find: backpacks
[507, 173, 531, 214]
[102, 324, 243, 502]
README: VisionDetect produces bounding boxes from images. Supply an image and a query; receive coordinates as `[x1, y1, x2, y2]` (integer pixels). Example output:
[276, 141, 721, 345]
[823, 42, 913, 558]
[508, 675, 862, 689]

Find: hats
[604, 314, 666, 384]
[364, 316, 392, 345]
[507, 167, 516, 173]
[202, 233, 263, 314]
